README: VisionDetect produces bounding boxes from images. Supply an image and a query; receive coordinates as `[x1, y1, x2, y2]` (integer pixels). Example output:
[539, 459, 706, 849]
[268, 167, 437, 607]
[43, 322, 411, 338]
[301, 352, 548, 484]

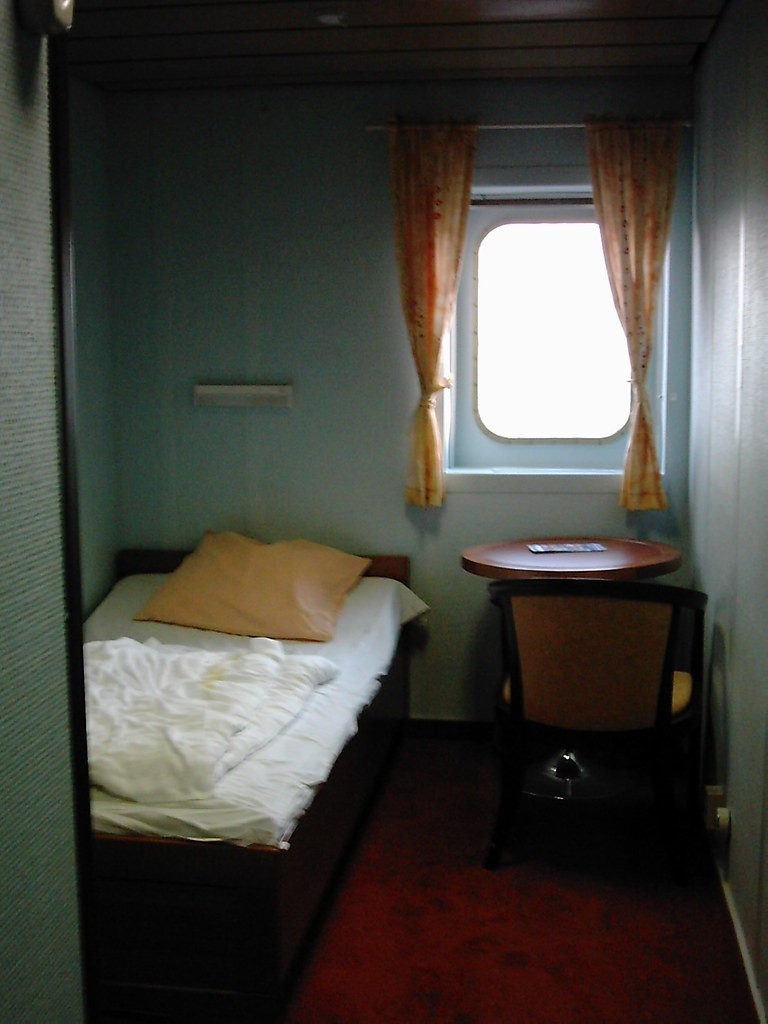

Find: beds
[81, 530, 431, 1024]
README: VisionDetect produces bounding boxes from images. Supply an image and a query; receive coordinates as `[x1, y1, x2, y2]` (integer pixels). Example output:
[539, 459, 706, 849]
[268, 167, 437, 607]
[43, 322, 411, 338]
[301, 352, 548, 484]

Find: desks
[459, 534, 683, 581]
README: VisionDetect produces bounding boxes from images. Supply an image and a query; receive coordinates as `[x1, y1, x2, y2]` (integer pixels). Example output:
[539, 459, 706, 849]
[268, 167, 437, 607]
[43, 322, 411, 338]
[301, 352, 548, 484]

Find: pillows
[132, 532, 371, 642]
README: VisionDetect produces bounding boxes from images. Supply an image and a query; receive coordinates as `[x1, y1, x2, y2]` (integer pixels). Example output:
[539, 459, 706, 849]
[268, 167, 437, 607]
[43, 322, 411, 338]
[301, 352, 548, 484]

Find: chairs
[482, 579, 704, 883]
